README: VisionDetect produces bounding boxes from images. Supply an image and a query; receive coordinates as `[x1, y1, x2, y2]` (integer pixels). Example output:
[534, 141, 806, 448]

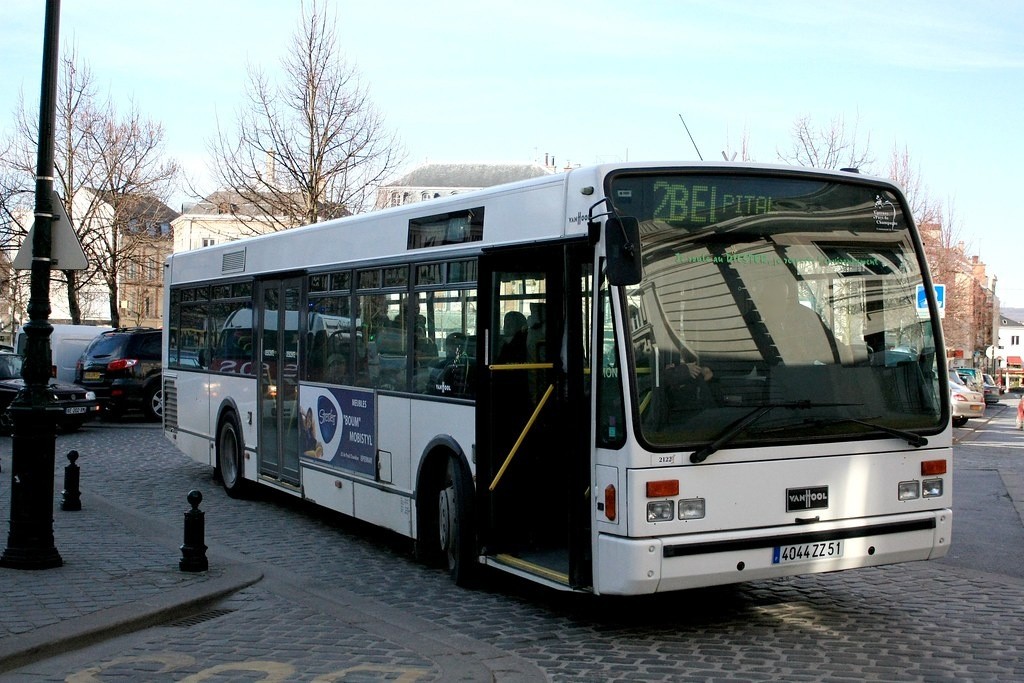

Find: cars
[0, 352, 88, 436]
[934, 367, 1001, 427]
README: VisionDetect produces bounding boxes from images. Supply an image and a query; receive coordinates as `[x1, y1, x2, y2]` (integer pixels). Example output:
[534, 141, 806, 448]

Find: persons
[732, 272, 862, 364]
[604, 303, 714, 430]
[324, 294, 476, 401]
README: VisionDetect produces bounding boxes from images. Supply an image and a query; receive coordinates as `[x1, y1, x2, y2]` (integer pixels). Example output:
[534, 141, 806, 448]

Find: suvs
[72, 325, 162, 425]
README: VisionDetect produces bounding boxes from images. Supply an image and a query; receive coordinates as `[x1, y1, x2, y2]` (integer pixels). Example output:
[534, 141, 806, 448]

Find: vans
[12, 324, 119, 386]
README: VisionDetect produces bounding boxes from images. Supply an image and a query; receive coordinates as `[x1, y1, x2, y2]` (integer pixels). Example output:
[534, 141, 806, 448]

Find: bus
[160, 162, 954, 596]
[212, 305, 373, 421]
